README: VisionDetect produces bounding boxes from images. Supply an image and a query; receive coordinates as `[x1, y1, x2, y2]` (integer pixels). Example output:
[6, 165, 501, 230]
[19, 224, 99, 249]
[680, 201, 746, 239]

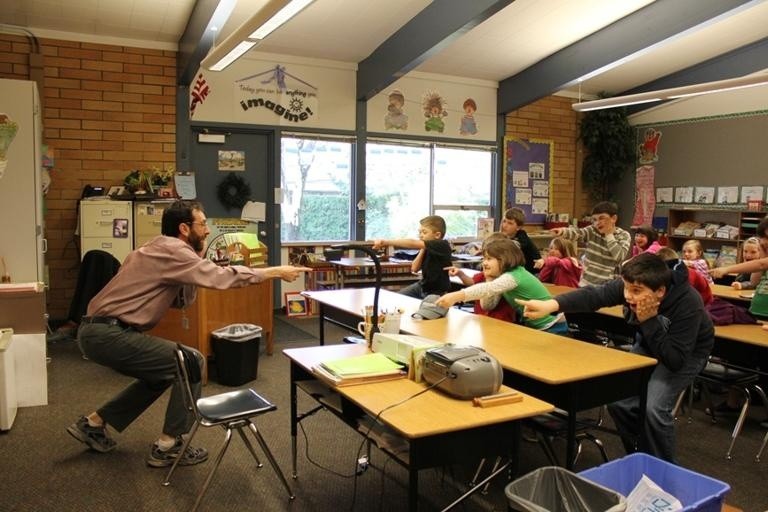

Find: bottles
[1, 276, 10, 283]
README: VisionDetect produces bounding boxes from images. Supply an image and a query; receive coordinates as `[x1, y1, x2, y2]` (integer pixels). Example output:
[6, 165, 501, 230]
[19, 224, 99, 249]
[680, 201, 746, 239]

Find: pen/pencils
[364, 305, 388, 324]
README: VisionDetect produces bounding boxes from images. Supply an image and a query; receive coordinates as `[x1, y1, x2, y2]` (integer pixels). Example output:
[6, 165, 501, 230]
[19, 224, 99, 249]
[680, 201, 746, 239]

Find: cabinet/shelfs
[77, 198, 133, 271]
[140, 263, 274, 387]
[132, 198, 176, 256]
[661, 207, 768, 263]
[0, 77, 51, 409]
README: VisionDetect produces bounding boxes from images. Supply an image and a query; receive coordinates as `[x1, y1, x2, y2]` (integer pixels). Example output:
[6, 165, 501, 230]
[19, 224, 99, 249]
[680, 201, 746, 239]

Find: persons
[513, 252, 715, 463]
[433, 232, 569, 441]
[66, 202, 312, 468]
[374, 216, 451, 299]
[533, 202, 631, 286]
[706, 218, 767, 415]
[500, 207, 543, 275]
[631, 227, 734, 392]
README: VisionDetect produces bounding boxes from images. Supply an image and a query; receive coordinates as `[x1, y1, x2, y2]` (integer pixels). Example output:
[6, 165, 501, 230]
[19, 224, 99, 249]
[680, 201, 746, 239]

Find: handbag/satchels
[705, 295, 756, 325]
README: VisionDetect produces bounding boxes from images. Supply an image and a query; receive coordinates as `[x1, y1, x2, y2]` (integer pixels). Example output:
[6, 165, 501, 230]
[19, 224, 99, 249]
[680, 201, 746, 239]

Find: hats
[411, 294, 449, 320]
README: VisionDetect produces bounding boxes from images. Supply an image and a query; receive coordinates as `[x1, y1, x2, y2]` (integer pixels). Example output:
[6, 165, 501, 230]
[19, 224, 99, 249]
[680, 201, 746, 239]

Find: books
[312, 352, 407, 387]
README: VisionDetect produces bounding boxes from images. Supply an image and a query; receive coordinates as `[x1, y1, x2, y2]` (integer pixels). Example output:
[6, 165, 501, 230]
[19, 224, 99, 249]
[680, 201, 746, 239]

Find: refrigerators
[0, 78, 49, 282]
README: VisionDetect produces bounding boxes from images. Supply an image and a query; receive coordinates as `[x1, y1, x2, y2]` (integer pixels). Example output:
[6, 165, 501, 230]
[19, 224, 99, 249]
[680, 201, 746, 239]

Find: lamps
[195, 1, 319, 73]
[570, 67, 767, 116]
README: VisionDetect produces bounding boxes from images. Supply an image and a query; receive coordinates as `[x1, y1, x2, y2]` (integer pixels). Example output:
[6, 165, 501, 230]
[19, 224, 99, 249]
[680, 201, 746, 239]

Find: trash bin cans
[505, 452, 732, 512]
[208, 323, 263, 386]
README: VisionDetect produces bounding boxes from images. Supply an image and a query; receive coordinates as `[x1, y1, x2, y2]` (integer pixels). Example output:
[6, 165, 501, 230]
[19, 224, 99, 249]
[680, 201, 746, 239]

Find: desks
[280, 260, 767, 510]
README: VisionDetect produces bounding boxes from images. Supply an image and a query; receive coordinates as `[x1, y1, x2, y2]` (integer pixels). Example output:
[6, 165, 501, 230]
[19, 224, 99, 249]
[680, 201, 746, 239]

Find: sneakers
[67, 415, 117, 453]
[148, 436, 208, 467]
[705, 399, 740, 416]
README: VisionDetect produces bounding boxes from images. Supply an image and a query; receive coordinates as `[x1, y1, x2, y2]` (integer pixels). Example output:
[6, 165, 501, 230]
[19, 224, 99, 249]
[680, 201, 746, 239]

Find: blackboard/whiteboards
[502, 135, 555, 226]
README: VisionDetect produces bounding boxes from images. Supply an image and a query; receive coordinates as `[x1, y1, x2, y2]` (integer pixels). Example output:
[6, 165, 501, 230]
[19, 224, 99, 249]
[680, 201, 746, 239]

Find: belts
[82, 316, 138, 331]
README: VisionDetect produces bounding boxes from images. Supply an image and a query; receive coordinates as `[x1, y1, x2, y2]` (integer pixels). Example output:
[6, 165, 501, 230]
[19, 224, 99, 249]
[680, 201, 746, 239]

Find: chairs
[160, 339, 296, 512]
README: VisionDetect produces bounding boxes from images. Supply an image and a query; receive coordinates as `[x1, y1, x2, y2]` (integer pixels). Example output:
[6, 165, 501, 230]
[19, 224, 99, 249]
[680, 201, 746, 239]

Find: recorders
[423, 343, 503, 400]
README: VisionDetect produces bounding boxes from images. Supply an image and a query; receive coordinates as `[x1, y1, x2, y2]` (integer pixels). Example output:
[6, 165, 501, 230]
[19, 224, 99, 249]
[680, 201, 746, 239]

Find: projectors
[371, 332, 445, 367]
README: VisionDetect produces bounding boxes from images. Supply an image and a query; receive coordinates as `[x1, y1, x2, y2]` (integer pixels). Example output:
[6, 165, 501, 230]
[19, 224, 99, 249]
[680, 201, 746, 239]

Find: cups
[356, 313, 403, 348]
[581, 215, 593, 223]
[568, 217, 579, 228]
